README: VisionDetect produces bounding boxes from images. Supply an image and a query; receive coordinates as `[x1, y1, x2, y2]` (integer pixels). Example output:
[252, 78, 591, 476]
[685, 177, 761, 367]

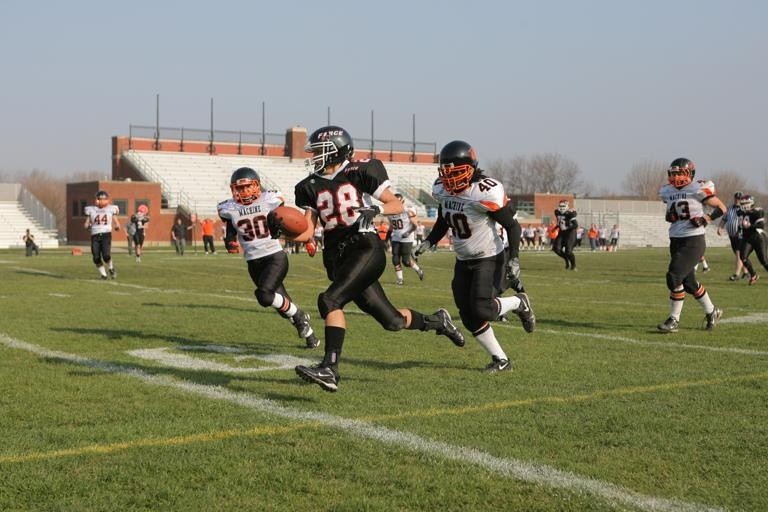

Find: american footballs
[267, 206, 308, 235]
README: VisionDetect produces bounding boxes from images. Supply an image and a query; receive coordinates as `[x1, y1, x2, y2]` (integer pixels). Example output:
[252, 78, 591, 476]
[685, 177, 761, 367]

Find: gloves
[667, 211, 677, 224]
[267, 211, 284, 239]
[414, 241, 432, 256]
[223, 237, 240, 253]
[505, 257, 520, 281]
[352, 205, 380, 229]
[689, 216, 707, 228]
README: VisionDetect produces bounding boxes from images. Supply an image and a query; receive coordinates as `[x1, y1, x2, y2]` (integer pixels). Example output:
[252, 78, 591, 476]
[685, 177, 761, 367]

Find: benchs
[0, 200, 59, 250]
[614, 213, 732, 249]
[577, 213, 669, 248]
[370, 180, 427, 217]
[122, 149, 233, 221]
[293, 157, 315, 173]
[358, 158, 441, 204]
[217, 152, 311, 216]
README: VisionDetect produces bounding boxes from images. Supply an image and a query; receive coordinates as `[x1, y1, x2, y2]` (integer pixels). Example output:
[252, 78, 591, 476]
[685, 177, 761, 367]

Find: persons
[378, 221, 454, 251]
[285, 224, 325, 253]
[657, 158, 728, 334]
[410, 140, 535, 376]
[202, 219, 226, 254]
[550, 199, 579, 271]
[576, 224, 620, 251]
[123, 210, 150, 262]
[268, 125, 465, 391]
[84, 191, 120, 280]
[22, 228, 38, 257]
[520, 223, 559, 250]
[216, 166, 320, 349]
[735, 194, 768, 285]
[382, 194, 423, 286]
[172, 218, 196, 255]
[717, 191, 750, 281]
[695, 256, 710, 272]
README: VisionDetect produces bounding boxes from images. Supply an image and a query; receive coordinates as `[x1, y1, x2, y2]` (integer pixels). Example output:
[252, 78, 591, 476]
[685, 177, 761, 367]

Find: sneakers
[703, 305, 724, 331]
[518, 244, 618, 253]
[657, 316, 680, 334]
[565, 259, 570, 269]
[695, 266, 759, 285]
[285, 242, 325, 254]
[108, 268, 117, 280]
[482, 280, 536, 375]
[305, 333, 321, 348]
[100, 275, 108, 281]
[392, 257, 423, 285]
[292, 309, 314, 339]
[128, 249, 219, 265]
[570, 263, 576, 269]
[433, 307, 465, 348]
[294, 361, 341, 392]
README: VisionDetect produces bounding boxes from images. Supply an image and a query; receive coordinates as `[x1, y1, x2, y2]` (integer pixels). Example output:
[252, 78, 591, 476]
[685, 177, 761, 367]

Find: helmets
[229, 167, 262, 206]
[304, 126, 355, 176]
[436, 140, 479, 195]
[95, 191, 110, 207]
[557, 200, 569, 215]
[740, 194, 755, 212]
[667, 158, 695, 190]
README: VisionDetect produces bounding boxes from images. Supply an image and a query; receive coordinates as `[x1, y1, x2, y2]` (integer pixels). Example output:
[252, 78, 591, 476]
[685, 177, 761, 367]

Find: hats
[734, 192, 742, 200]
